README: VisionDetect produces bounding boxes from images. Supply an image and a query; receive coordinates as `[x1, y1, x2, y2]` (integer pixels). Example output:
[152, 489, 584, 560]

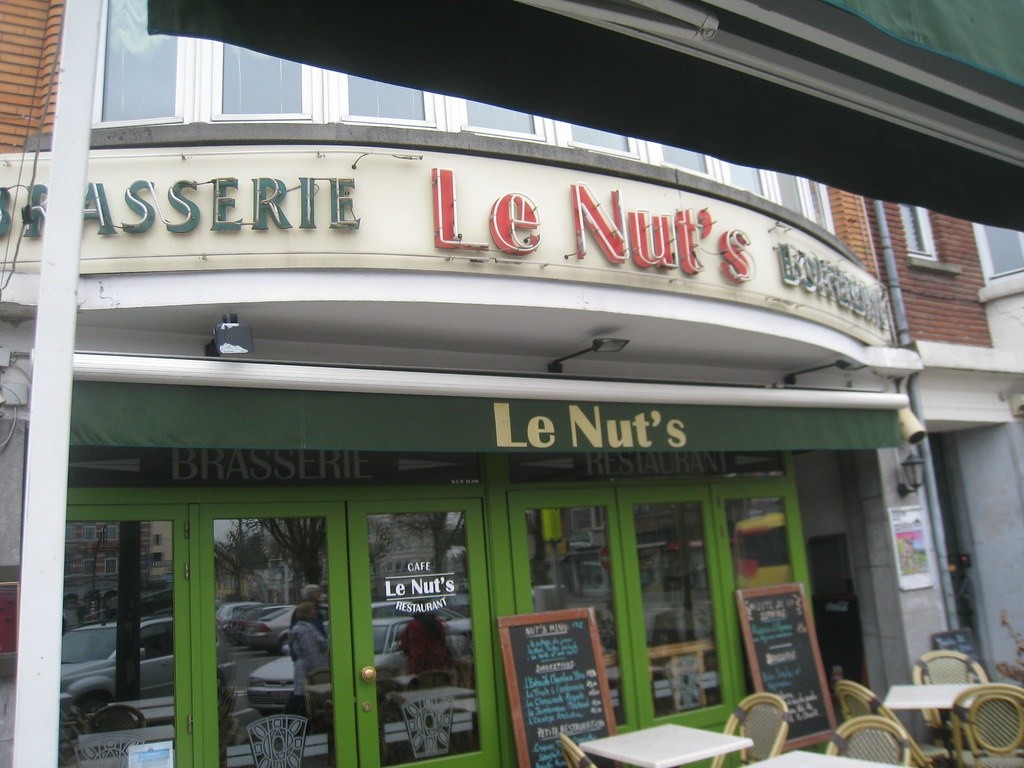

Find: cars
[247, 616, 474, 721]
[215, 602, 329, 656]
[371, 600, 473, 636]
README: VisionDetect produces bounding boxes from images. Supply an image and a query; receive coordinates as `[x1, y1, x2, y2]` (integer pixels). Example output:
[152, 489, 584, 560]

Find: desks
[738, 748, 917, 768]
[72, 724, 175, 762]
[579, 722, 755, 768]
[390, 685, 479, 751]
[878, 682, 1024, 760]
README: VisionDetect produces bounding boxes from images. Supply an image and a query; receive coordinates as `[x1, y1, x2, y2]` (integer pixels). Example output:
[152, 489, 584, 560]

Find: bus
[735, 513, 794, 588]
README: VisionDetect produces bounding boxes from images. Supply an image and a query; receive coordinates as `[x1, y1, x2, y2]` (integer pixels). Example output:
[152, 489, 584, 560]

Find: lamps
[896, 451, 925, 497]
[204, 312, 254, 359]
[547, 335, 629, 374]
[783, 358, 863, 384]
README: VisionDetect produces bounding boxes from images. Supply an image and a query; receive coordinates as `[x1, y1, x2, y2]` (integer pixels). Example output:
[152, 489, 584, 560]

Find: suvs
[60, 614, 233, 714]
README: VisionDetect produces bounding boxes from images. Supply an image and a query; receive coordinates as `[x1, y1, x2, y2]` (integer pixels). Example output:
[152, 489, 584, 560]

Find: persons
[287, 585, 331, 735]
[397, 600, 452, 677]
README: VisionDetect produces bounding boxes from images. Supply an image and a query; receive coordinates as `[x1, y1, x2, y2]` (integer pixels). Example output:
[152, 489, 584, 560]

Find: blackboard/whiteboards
[736, 583, 837, 752]
[497, 607, 624, 768]
[933, 627, 992, 682]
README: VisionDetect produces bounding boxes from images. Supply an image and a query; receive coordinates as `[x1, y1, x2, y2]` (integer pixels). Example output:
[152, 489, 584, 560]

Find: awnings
[72, 348, 911, 453]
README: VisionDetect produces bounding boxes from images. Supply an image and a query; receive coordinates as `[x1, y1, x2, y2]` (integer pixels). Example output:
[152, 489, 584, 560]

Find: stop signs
[599, 546, 610, 571]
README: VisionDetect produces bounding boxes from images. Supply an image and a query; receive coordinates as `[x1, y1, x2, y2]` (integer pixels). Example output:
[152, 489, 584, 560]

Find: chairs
[58, 659, 482, 768]
[557, 648, 1024, 768]
